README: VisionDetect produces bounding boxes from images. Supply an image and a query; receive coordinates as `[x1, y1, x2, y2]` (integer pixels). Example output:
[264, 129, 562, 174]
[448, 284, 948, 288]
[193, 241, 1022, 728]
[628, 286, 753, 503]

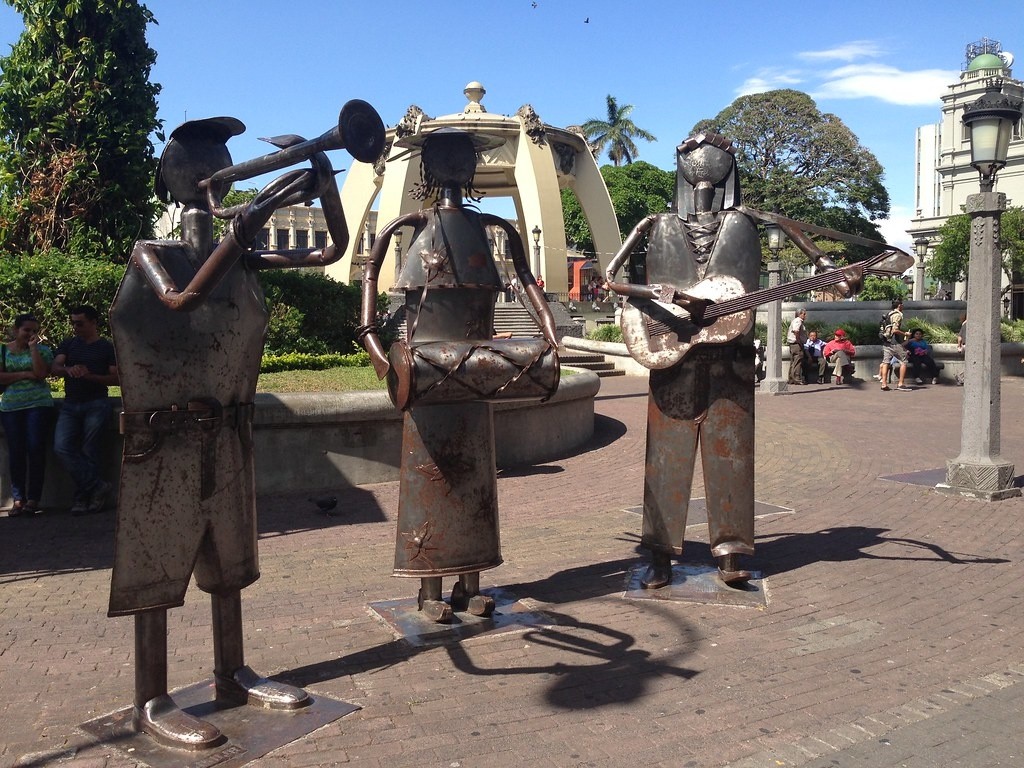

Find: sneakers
[71, 480, 113, 515]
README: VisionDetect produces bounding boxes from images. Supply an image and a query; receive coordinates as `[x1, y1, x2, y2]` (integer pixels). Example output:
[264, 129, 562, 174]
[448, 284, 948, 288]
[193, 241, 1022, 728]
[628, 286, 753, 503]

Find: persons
[804, 331, 829, 384]
[507, 274, 517, 303]
[787, 309, 806, 386]
[955, 319, 966, 384]
[823, 329, 855, 385]
[881, 297, 912, 392]
[51, 304, 121, 516]
[536, 275, 544, 291]
[361, 128, 562, 625]
[109, 114, 348, 753]
[606, 131, 865, 593]
[902, 328, 938, 385]
[872, 356, 898, 383]
[588, 276, 610, 310]
[0, 313, 54, 518]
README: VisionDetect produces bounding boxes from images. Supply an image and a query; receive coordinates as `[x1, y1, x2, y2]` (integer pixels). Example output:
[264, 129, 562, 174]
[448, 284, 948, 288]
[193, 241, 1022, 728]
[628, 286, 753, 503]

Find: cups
[904, 331, 909, 340]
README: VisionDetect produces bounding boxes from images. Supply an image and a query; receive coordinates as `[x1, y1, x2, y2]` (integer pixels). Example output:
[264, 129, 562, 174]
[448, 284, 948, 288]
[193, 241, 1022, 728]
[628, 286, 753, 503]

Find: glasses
[71, 320, 88, 327]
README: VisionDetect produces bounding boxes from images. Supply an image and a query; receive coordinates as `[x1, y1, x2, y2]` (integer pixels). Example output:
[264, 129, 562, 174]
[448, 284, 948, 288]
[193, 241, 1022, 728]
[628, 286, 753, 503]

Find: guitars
[620, 249, 916, 370]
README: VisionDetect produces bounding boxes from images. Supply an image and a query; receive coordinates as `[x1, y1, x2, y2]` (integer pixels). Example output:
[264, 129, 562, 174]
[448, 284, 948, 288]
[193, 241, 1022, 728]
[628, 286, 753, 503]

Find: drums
[387, 338, 560, 413]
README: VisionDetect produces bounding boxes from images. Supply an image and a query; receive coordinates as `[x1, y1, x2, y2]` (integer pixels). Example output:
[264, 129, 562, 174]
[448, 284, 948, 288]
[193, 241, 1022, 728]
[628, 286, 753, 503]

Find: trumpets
[196, 99, 387, 220]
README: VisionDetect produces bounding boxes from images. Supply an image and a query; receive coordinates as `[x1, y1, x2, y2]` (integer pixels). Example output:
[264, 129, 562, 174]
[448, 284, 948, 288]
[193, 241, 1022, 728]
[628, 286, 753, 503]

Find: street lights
[532, 225, 542, 280]
[760, 202, 792, 395]
[935, 77, 1023, 502]
[1002, 297, 1010, 317]
[913, 237, 929, 301]
[393, 228, 403, 285]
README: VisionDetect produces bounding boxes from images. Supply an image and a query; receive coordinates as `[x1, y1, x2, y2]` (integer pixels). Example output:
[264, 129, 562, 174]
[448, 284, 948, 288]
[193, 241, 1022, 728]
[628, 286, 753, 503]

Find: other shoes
[8, 498, 47, 516]
[795, 381, 808, 385]
[881, 385, 893, 391]
[873, 374, 883, 382]
[932, 377, 937, 384]
[896, 384, 912, 391]
[816, 378, 823, 384]
[915, 377, 922, 383]
[836, 375, 841, 385]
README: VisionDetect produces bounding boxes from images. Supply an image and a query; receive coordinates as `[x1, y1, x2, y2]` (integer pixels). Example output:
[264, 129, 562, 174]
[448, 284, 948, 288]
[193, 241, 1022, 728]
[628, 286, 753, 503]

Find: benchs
[807, 345, 855, 383]
[887, 345, 944, 384]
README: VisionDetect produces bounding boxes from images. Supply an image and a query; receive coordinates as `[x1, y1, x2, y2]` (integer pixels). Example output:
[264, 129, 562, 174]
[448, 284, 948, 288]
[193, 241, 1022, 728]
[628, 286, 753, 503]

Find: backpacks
[878, 311, 898, 343]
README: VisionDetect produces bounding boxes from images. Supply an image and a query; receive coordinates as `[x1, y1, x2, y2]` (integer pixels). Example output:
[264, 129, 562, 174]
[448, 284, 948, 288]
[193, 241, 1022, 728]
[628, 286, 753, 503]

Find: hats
[834, 329, 846, 336]
